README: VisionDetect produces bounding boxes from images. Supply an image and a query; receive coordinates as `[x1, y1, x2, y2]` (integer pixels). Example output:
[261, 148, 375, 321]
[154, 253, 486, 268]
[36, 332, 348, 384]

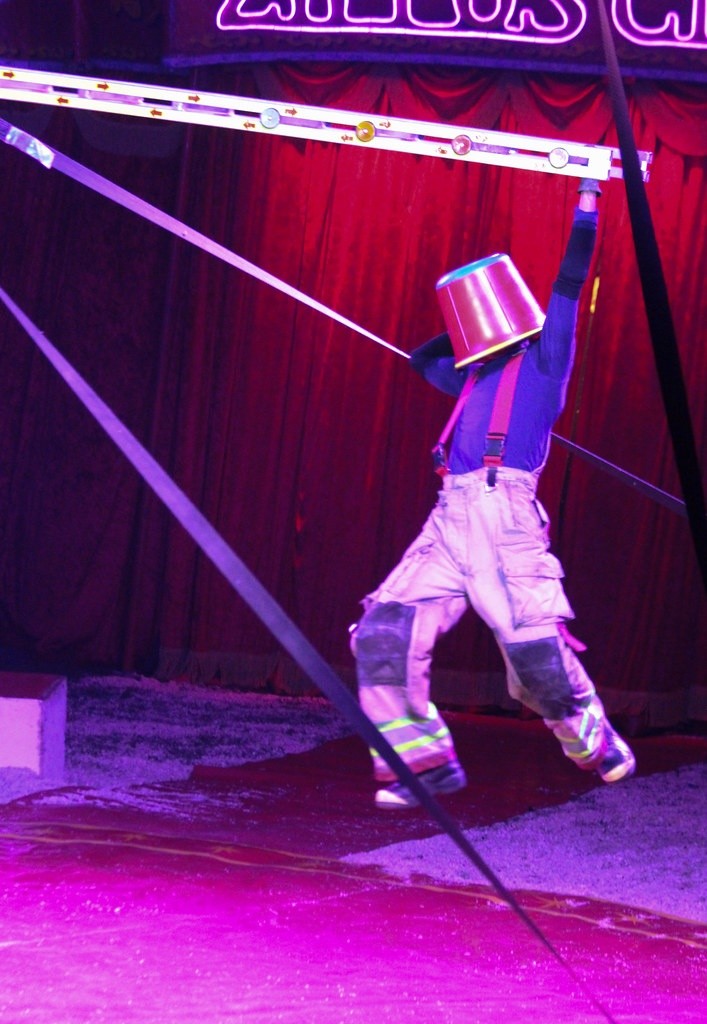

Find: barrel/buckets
[435, 253, 546, 369]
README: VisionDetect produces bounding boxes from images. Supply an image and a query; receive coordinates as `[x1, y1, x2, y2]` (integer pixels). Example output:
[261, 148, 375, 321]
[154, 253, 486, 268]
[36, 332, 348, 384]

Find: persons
[349, 177, 634, 807]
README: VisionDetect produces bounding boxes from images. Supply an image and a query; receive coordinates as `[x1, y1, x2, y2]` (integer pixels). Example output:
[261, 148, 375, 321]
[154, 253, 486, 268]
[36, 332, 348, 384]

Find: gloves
[577, 145, 602, 196]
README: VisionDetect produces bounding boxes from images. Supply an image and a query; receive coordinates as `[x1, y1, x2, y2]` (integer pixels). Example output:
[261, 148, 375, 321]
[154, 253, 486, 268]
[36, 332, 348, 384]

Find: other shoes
[375, 760, 467, 805]
[595, 718, 636, 783]
